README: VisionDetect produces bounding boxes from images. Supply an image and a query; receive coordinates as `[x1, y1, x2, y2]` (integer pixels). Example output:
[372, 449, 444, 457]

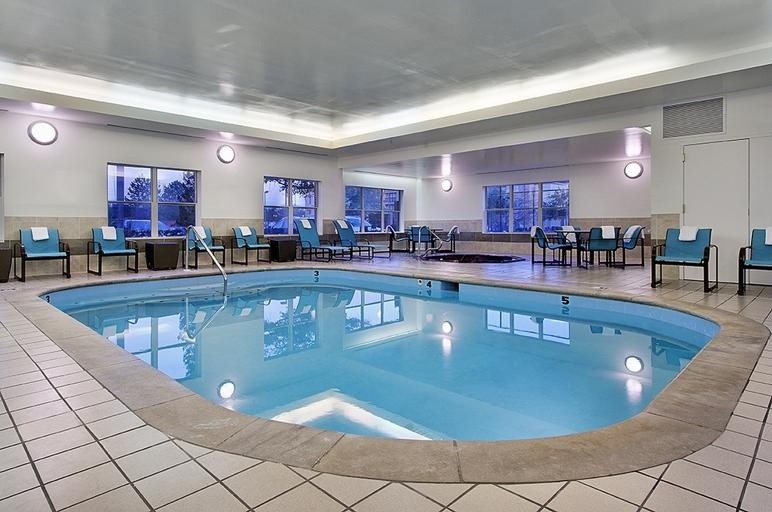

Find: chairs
[333, 218, 375, 260]
[294, 219, 353, 262]
[385, 222, 460, 258]
[184, 225, 229, 268]
[82, 281, 362, 344]
[651, 228, 719, 292]
[13, 226, 71, 280]
[86, 226, 140, 276]
[529, 312, 702, 377]
[230, 226, 273, 266]
[736, 229, 772, 295]
[527, 223, 646, 270]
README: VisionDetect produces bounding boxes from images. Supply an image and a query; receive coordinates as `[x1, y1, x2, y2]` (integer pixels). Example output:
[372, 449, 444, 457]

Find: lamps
[27, 119, 59, 145]
[217, 144, 236, 164]
[441, 179, 453, 193]
[218, 380, 238, 399]
[623, 160, 643, 178]
[440, 319, 454, 335]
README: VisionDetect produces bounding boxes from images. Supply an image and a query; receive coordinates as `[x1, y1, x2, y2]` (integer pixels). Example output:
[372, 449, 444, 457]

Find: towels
[101, 226, 117, 242]
[30, 226, 51, 242]
[765, 229, 772, 246]
[337, 219, 349, 229]
[192, 226, 207, 241]
[300, 219, 312, 230]
[239, 226, 253, 236]
[678, 227, 699, 243]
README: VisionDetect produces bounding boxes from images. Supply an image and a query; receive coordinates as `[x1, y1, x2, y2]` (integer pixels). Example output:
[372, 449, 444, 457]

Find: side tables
[267, 237, 298, 263]
[143, 240, 180, 270]
